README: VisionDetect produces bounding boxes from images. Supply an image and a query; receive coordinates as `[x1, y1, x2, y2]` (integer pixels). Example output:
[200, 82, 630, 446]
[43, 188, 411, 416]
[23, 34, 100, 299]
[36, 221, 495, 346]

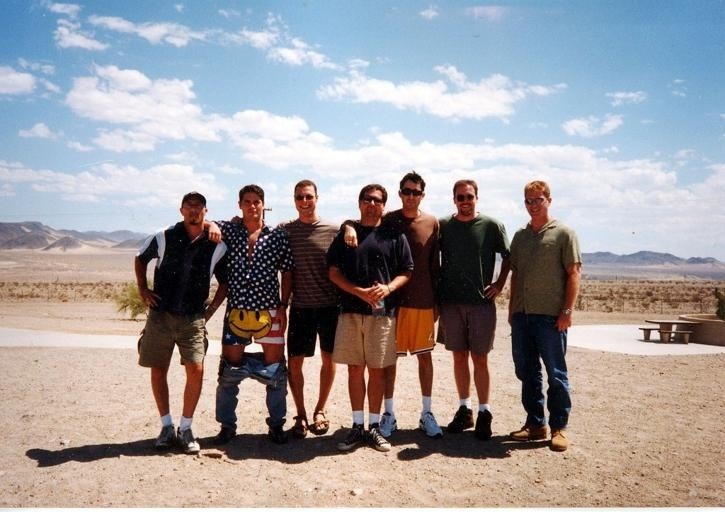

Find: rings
[350, 240, 352, 244]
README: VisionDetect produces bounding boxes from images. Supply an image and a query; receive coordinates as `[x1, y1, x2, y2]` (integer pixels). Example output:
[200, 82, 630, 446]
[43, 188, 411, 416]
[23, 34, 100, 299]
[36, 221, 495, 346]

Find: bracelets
[279, 303, 288, 307]
[387, 285, 392, 294]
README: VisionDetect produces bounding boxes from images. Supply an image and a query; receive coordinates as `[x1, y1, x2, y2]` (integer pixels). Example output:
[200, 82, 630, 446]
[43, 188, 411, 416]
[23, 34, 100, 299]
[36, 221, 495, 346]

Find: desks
[646, 320, 702, 343]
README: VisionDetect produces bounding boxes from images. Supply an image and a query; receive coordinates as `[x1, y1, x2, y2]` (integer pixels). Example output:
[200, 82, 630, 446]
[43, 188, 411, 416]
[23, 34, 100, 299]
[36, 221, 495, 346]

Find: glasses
[457, 194, 474, 201]
[525, 198, 544, 204]
[295, 195, 313, 200]
[401, 187, 422, 196]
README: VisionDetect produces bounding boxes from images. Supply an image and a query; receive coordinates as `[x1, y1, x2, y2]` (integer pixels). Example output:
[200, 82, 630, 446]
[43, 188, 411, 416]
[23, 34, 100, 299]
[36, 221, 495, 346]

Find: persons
[134, 191, 229, 455]
[325, 184, 415, 452]
[432, 178, 512, 443]
[507, 179, 583, 450]
[227, 178, 342, 439]
[338, 170, 444, 441]
[202, 184, 298, 445]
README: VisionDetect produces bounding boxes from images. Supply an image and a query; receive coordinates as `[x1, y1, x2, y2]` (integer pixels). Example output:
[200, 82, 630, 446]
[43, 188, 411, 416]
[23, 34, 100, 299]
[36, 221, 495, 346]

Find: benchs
[659, 330, 696, 344]
[639, 327, 659, 341]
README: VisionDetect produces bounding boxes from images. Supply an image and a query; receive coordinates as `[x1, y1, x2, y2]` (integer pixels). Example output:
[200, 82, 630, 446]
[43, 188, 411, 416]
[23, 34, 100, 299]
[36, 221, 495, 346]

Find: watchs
[562, 307, 573, 315]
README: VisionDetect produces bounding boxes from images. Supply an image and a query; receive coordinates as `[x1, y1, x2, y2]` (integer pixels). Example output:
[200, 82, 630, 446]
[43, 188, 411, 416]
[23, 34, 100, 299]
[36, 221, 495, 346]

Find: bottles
[371, 280, 385, 320]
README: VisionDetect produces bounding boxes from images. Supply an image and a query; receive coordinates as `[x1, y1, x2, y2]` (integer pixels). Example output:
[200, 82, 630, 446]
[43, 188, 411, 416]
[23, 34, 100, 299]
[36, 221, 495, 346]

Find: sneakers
[551, 430, 568, 450]
[156, 424, 176, 448]
[268, 429, 288, 444]
[337, 412, 397, 451]
[510, 424, 547, 441]
[177, 427, 200, 454]
[420, 404, 492, 438]
[214, 428, 233, 445]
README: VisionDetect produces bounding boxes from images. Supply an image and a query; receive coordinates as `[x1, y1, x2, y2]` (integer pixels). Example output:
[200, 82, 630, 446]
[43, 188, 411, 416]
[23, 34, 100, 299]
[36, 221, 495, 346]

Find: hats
[182, 191, 206, 205]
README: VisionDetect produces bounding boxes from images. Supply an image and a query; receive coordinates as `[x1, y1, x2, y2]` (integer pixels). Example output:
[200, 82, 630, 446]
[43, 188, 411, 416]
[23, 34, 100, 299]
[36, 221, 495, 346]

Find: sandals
[313, 411, 329, 434]
[292, 416, 308, 438]
